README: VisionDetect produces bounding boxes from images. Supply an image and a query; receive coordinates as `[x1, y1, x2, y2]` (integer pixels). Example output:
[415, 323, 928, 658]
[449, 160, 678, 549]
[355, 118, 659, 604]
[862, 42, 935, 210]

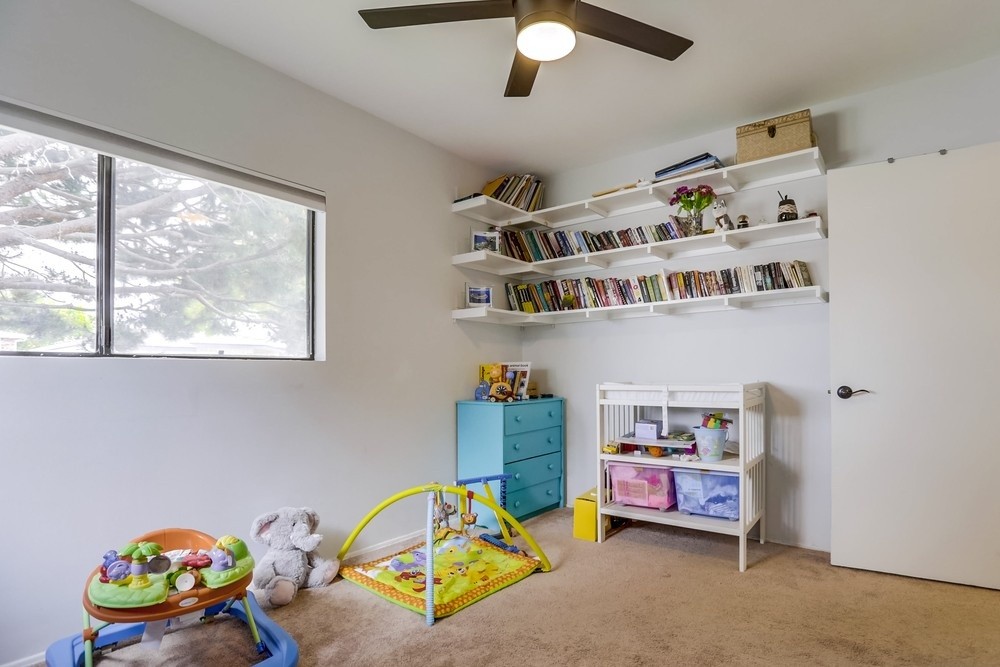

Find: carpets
[337, 525, 542, 619]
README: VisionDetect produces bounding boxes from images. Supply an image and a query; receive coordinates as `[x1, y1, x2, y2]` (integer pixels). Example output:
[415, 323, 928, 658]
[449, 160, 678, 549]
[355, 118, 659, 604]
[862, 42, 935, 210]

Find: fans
[358, 0, 694, 98]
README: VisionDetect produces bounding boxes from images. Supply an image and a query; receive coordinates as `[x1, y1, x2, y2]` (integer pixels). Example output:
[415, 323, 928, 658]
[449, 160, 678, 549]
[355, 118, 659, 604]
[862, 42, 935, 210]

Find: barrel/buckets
[692, 426, 729, 462]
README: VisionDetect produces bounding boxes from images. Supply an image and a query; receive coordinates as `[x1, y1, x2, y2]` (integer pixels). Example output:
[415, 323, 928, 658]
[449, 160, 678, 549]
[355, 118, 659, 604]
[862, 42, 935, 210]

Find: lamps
[516, 11, 577, 62]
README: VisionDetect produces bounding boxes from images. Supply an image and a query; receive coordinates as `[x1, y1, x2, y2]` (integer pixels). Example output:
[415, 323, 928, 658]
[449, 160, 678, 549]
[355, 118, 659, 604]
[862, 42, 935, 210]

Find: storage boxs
[634, 419, 662, 439]
[736, 108, 814, 163]
[606, 461, 676, 513]
[669, 467, 739, 521]
[572, 486, 618, 544]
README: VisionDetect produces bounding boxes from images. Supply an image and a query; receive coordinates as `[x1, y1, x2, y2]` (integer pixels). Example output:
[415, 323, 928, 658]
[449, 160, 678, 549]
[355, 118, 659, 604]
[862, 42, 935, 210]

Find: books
[504, 215, 702, 263]
[452, 173, 544, 212]
[504, 260, 812, 314]
[652, 153, 726, 184]
[479, 362, 530, 395]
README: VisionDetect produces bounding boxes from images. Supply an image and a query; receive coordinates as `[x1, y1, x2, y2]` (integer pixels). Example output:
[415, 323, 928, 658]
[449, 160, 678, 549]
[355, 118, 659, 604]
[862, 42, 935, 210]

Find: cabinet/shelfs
[595, 382, 765, 574]
[456, 396, 565, 533]
[449, 144, 829, 326]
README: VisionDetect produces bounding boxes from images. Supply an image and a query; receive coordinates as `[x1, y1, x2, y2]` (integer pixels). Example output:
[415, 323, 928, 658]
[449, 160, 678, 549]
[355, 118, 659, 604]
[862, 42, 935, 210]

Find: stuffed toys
[252, 507, 340, 608]
